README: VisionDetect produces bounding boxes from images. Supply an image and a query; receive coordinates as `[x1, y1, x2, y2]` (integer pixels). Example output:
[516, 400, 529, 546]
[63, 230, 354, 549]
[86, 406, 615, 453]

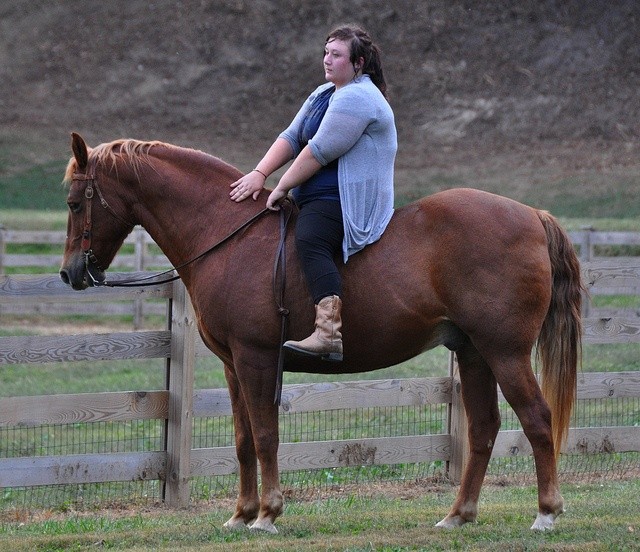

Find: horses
[58, 131, 594, 536]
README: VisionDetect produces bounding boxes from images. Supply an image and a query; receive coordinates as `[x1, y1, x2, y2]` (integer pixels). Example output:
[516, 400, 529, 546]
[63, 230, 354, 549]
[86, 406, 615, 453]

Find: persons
[229, 24, 398, 364]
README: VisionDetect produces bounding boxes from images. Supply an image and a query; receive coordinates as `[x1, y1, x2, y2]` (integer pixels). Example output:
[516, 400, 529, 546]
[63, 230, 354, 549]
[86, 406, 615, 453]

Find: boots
[282, 295, 346, 363]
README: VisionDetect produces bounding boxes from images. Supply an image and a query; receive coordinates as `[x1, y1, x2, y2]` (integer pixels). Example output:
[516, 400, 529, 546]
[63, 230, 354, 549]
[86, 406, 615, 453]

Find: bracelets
[253, 169, 267, 180]
